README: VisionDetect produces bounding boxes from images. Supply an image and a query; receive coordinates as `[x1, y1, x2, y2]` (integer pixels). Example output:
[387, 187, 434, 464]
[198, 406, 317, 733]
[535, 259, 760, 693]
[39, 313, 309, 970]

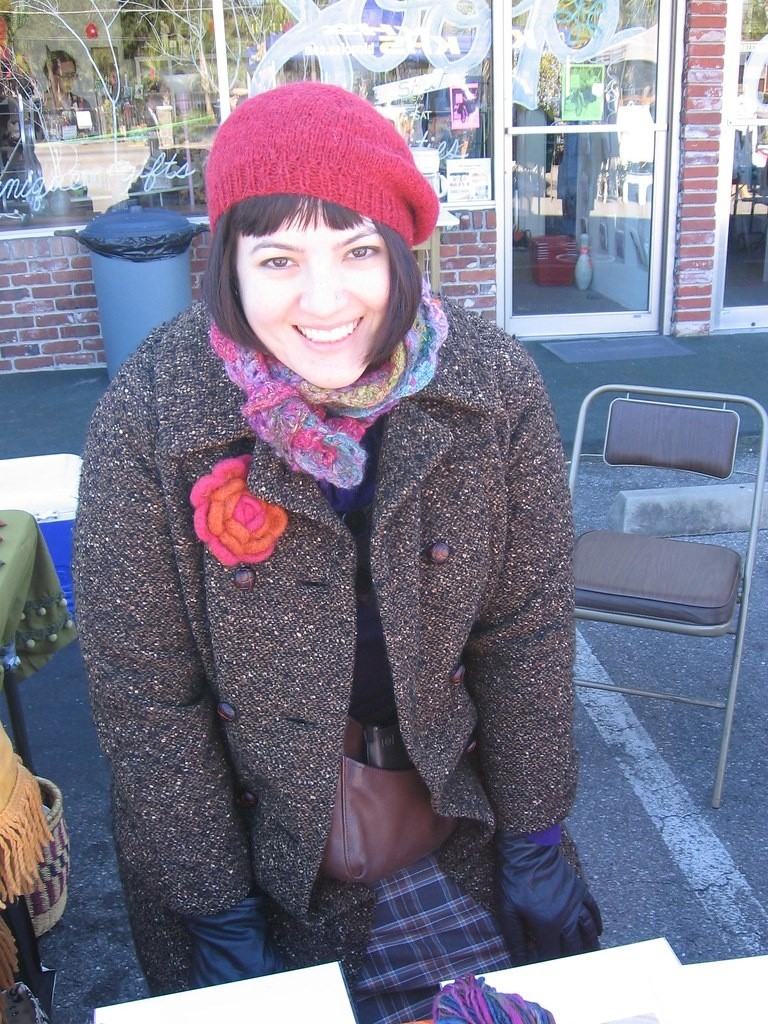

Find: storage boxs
[531, 233, 579, 287]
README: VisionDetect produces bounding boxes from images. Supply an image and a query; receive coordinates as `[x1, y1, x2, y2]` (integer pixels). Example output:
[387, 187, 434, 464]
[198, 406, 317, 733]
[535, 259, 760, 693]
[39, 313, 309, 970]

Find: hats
[205, 82, 439, 249]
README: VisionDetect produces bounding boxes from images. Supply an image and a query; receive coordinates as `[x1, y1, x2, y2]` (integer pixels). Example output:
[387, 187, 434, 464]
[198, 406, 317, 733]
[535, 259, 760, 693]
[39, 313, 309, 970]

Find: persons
[72, 81, 603, 1024]
[27, 50, 98, 143]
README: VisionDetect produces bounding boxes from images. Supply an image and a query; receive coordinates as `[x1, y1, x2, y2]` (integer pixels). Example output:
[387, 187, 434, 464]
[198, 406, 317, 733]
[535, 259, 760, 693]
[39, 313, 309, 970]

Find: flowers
[191, 454, 289, 565]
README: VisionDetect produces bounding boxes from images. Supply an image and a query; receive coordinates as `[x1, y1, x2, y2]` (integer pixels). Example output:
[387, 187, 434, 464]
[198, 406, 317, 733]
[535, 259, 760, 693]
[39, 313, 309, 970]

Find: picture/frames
[92, 46, 122, 96]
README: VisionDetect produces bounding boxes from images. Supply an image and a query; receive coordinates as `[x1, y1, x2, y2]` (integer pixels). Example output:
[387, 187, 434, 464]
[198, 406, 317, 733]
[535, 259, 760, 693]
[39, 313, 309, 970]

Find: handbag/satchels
[320, 715, 459, 882]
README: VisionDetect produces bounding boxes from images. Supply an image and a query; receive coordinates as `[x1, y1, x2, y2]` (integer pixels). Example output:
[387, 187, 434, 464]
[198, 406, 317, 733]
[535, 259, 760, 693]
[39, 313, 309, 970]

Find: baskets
[24, 775, 70, 937]
[529, 237, 578, 287]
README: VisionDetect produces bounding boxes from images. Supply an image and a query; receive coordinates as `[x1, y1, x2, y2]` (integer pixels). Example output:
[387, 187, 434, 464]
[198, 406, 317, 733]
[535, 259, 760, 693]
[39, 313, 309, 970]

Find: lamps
[163, 73, 200, 207]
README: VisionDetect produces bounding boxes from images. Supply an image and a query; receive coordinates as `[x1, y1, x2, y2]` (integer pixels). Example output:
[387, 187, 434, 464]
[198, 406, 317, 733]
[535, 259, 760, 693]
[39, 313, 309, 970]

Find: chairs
[566, 383, 768, 807]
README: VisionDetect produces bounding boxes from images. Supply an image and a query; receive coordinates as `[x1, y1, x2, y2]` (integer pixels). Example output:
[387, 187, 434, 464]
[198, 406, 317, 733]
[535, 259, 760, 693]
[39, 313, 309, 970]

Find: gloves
[181, 897, 284, 989]
[494, 833, 602, 967]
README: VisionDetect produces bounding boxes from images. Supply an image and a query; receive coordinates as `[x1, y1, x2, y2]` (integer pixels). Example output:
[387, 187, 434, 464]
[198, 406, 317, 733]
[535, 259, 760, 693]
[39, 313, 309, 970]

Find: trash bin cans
[54, 205, 212, 383]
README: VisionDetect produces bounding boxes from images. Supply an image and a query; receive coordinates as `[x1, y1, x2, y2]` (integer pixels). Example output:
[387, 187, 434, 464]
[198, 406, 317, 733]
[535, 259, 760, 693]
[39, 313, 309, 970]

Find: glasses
[55, 72, 78, 81]
